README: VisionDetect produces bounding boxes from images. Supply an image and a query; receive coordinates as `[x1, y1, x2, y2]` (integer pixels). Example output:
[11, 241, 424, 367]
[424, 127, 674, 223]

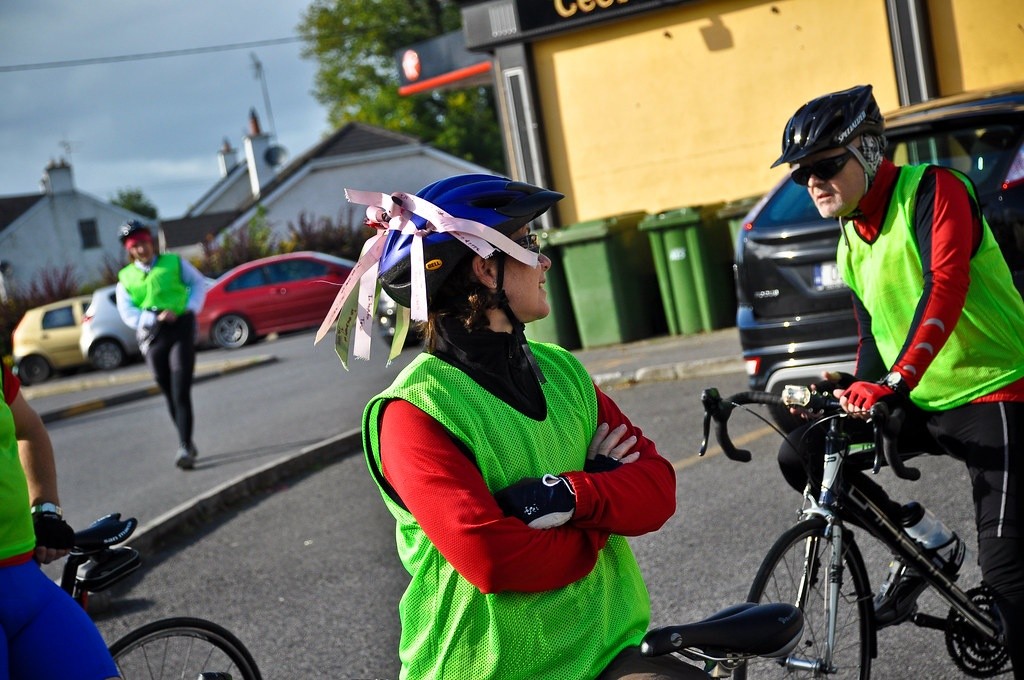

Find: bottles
[898, 501, 967, 569]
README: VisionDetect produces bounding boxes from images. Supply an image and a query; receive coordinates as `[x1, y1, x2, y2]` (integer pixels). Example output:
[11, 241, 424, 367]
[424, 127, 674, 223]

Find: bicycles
[698, 384, 1015, 680]
[60, 512, 263, 680]
[600, 601, 804, 680]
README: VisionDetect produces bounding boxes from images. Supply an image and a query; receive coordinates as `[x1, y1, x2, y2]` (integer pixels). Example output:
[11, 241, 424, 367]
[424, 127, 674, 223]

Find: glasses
[790, 146, 864, 186]
[496, 232, 541, 255]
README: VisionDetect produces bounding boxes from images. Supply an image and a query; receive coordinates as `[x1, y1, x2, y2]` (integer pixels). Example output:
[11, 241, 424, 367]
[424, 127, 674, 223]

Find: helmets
[377, 175, 565, 310]
[118, 220, 151, 244]
[770, 85, 884, 168]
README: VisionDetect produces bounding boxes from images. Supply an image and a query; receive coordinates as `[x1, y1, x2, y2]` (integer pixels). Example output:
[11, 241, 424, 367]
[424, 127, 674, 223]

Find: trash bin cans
[525, 228, 583, 348]
[717, 194, 771, 256]
[549, 210, 671, 350]
[638, 201, 737, 336]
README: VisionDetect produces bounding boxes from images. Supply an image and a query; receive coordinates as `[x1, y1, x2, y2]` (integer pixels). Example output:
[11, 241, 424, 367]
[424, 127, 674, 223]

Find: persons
[114, 217, 208, 471]
[0, 348, 124, 680]
[315, 173, 712, 680]
[765, 84, 1024, 680]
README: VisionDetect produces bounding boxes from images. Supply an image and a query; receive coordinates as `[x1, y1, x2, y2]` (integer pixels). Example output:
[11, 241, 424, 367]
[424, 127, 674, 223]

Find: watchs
[29, 502, 63, 518]
[880, 371, 910, 395]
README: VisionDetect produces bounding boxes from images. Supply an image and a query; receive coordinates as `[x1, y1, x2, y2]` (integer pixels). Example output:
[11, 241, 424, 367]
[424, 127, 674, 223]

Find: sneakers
[873, 556, 929, 630]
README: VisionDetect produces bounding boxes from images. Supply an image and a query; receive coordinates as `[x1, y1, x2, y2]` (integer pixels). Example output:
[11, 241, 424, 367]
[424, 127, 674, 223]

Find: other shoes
[175, 443, 198, 470]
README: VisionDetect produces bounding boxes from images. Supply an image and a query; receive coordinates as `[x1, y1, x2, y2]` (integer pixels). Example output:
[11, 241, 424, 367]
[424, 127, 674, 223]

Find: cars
[10, 296, 91, 386]
[195, 251, 356, 351]
[734, 84, 1024, 435]
[76, 274, 217, 370]
